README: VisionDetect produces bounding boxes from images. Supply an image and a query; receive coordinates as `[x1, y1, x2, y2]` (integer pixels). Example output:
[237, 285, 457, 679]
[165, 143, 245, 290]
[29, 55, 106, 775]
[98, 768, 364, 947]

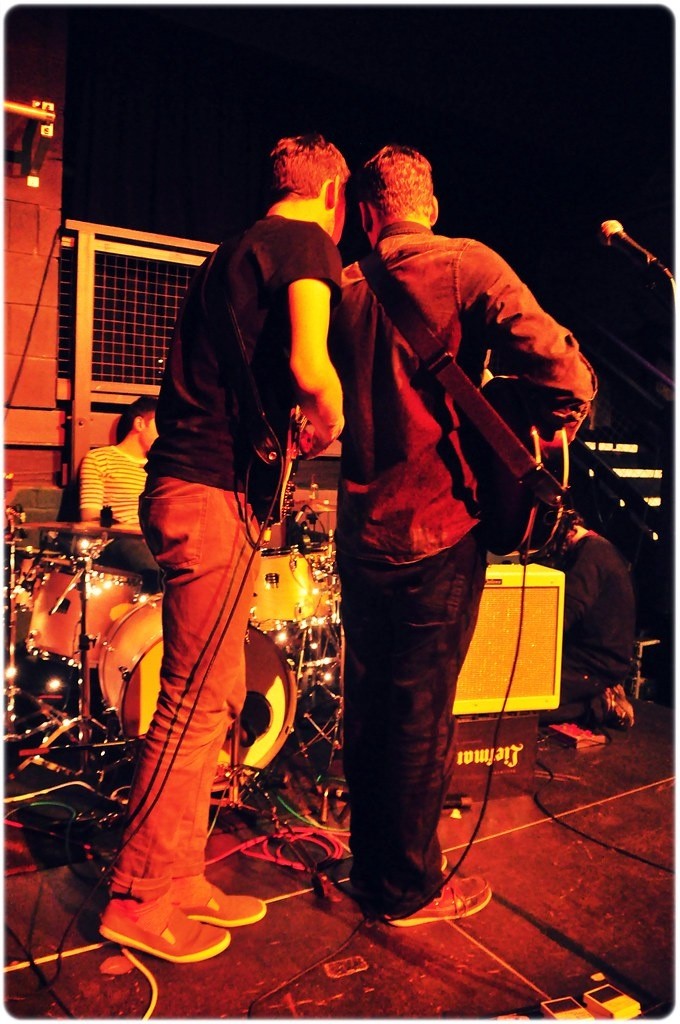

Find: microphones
[286, 506, 308, 532]
[597, 219, 673, 281]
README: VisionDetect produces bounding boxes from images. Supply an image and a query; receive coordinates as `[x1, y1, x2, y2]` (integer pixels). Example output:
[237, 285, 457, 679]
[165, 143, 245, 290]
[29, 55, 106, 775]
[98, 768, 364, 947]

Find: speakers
[452, 561, 566, 716]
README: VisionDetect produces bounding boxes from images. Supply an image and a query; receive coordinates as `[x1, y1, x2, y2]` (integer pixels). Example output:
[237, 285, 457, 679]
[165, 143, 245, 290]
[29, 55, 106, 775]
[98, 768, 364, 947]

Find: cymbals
[15, 520, 144, 541]
[283, 496, 342, 514]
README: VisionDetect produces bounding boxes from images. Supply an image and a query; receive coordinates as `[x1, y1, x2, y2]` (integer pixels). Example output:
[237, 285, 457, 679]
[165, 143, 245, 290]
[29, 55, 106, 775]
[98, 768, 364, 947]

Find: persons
[544, 508, 636, 729]
[80, 396, 164, 586]
[333, 145, 599, 925]
[99, 131, 350, 963]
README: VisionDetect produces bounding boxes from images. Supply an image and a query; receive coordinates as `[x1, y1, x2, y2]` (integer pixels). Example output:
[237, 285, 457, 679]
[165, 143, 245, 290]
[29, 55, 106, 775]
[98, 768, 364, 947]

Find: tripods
[4, 529, 349, 907]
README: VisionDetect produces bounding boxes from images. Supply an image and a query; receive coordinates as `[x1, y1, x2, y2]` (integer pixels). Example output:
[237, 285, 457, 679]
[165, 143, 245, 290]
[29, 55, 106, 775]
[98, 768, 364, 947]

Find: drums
[6, 558, 145, 675]
[97, 590, 296, 788]
[241, 541, 345, 628]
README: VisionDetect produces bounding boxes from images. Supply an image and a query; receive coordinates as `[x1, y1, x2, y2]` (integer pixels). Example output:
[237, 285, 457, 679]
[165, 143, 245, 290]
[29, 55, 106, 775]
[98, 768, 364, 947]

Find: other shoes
[99, 901, 230, 963]
[609, 684, 635, 728]
[176, 883, 267, 928]
[385, 876, 491, 927]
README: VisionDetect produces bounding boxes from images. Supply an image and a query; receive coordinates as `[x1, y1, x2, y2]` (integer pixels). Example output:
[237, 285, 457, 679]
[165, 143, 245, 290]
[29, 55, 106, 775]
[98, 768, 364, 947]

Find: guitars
[461, 376, 571, 565]
[244, 397, 302, 526]
